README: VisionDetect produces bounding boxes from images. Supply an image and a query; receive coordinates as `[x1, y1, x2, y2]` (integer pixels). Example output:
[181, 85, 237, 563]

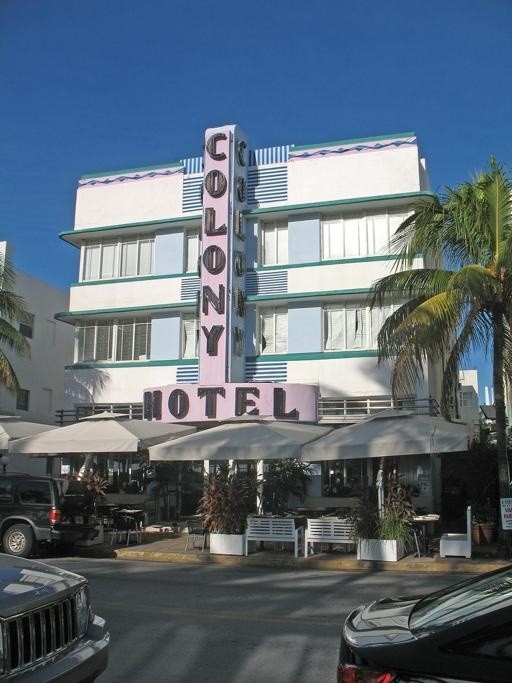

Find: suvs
[0, 470, 101, 558]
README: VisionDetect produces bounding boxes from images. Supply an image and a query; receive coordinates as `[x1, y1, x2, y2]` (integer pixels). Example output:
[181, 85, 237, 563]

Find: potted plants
[352, 497, 408, 563]
[196, 462, 268, 555]
[472, 498, 496, 546]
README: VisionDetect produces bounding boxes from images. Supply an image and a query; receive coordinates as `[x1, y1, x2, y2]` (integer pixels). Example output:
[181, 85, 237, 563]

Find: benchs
[245, 517, 303, 558]
[304, 518, 359, 559]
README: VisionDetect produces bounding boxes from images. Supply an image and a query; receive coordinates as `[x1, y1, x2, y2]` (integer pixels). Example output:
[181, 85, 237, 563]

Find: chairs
[97, 503, 145, 547]
[184, 521, 207, 553]
[440, 505, 472, 559]
[416, 511, 427, 516]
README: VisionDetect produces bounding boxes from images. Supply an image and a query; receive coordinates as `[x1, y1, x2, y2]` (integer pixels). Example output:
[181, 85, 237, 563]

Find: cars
[0, 552, 113, 682]
[334, 562, 511, 682]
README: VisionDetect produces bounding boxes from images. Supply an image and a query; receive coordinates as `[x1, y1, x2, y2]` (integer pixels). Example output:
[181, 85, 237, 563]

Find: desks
[274, 505, 353, 527]
[409, 514, 440, 558]
